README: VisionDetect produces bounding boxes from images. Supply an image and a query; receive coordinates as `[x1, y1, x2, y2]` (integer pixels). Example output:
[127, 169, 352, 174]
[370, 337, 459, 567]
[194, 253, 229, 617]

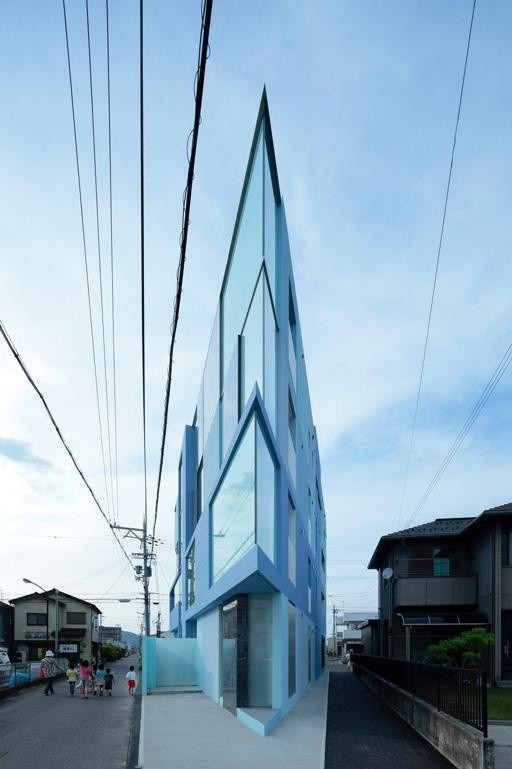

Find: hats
[46, 651, 53, 657]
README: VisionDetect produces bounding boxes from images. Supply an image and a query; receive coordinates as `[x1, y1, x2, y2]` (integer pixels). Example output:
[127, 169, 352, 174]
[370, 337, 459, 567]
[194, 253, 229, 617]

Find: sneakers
[84, 691, 111, 698]
[43, 691, 56, 696]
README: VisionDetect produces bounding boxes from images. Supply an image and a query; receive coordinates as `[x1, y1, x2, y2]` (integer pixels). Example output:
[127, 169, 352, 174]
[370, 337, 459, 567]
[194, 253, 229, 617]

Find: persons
[126, 666, 136, 696]
[66, 647, 115, 699]
[40, 651, 66, 695]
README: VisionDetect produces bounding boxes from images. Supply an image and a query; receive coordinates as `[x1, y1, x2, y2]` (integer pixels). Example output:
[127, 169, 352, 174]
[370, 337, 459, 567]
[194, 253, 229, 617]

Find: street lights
[120, 598, 150, 635]
[22, 577, 48, 651]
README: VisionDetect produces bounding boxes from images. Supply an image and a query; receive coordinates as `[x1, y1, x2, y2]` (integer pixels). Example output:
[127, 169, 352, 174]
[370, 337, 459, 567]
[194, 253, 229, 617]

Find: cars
[0, 647, 11, 681]
